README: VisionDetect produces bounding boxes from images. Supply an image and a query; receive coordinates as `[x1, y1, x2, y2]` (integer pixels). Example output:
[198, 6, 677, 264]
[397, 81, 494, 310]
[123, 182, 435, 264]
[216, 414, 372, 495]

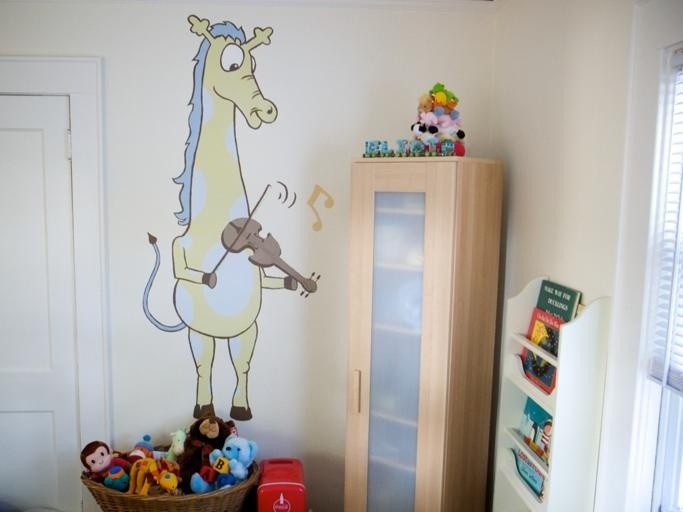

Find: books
[510, 396, 554, 504]
[518, 279, 582, 397]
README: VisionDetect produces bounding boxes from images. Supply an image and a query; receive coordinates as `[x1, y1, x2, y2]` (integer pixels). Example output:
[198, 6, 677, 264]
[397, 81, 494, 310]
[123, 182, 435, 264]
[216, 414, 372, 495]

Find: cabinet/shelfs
[343, 157, 506, 512]
[493, 276, 614, 512]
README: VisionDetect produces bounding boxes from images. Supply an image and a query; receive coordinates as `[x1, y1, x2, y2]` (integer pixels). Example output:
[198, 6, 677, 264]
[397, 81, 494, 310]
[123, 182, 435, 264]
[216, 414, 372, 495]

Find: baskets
[79, 459, 261, 511]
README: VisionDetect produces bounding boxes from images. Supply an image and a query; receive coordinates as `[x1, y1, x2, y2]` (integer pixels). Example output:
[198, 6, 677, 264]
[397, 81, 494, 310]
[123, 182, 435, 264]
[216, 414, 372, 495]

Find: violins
[221, 217, 321, 298]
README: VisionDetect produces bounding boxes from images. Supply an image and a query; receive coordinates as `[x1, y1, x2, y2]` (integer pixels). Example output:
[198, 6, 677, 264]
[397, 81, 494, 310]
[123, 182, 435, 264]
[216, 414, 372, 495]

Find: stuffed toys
[79, 439, 130, 479]
[410, 82, 465, 157]
[177, 412, 239, 490]
[104, 464, 129, 490]
[190, 433, 258, 494]
[169, 428, 186, 457]
[128, 458, 180, 497]
[127, 433, 154, 458]
